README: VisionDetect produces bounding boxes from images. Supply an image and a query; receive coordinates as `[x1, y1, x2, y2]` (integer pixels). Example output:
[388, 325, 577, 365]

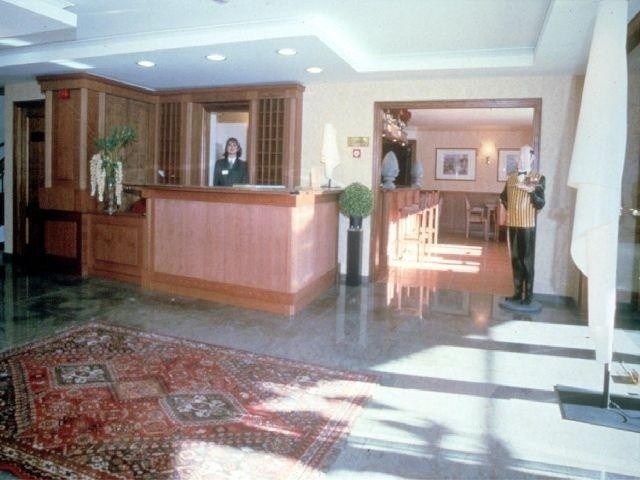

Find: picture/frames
[497, 148, 520, 182]
[435, 148, 476, 181]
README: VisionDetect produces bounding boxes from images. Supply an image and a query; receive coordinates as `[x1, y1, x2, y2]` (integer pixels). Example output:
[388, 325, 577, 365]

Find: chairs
[464, 195, 507, 242]
[396, 190, 440, 262]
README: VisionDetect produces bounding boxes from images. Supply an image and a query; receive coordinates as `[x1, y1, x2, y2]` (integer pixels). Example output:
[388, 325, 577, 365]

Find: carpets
[0, 322, 381, 480]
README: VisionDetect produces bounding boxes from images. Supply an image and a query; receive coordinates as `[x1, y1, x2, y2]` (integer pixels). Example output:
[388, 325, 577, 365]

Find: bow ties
[518, 171, 527, 176]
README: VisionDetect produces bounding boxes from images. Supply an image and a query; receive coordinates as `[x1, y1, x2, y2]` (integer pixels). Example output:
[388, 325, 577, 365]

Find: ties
[229, 160, 233, 168]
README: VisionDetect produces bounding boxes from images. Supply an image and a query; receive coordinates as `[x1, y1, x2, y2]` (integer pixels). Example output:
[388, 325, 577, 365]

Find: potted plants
[93, 125, 138, 216]
[336, 182, 375, 229]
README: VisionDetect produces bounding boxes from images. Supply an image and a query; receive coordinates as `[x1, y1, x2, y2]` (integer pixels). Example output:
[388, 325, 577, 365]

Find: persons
[497, 143, 546, 306]
[213, 137, 250, 186]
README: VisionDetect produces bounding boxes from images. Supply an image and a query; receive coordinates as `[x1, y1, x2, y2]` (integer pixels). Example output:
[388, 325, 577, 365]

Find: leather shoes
[505, 295, 533, 307]
